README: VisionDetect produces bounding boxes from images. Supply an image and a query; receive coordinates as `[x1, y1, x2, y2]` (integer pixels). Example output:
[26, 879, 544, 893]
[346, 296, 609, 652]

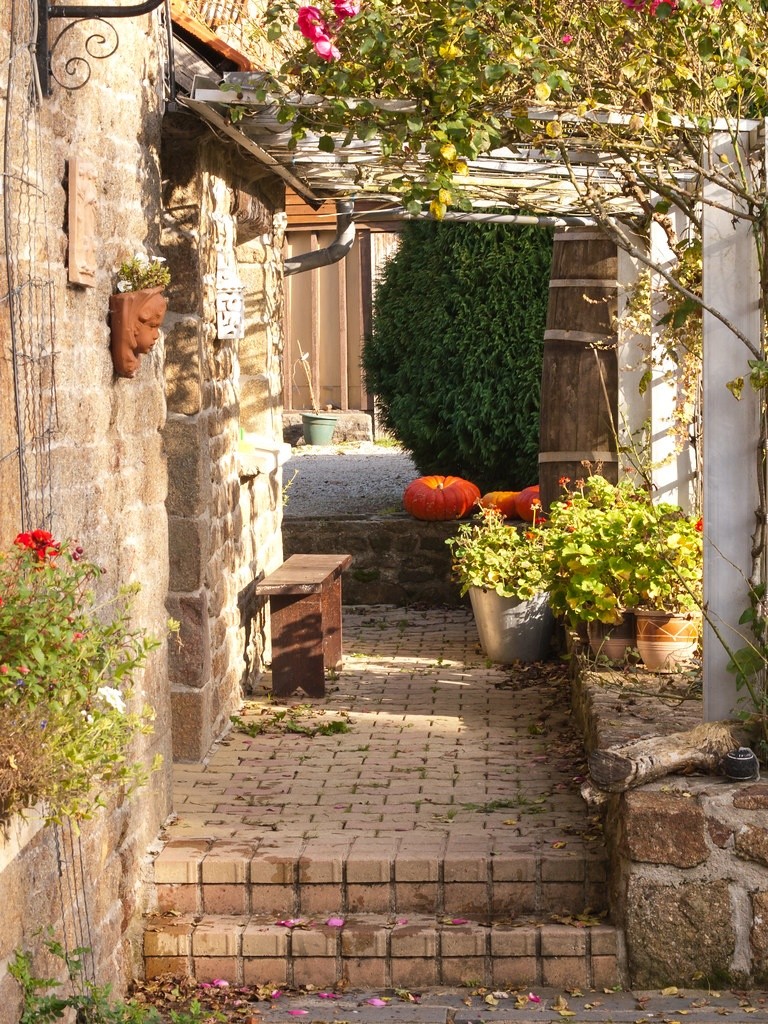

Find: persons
[133, 292, 167, 357]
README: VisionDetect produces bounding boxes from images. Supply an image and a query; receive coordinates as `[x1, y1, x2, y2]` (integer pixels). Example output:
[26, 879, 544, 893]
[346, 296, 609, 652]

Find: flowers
[113, 252, 171, 294]
[1, 528, 180, 831]
[446, 459, 705, 626]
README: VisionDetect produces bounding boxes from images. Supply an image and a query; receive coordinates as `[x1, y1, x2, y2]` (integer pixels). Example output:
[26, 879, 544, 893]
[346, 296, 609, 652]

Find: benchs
[256, 554, 352, 698]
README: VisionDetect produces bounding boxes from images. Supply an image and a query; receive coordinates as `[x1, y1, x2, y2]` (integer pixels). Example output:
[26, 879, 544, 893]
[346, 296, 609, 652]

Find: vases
[467, 585, 553, 664]
[634, 603, 701, 672]
[299, 413, 339, 445]
[587, 608, 642, 667]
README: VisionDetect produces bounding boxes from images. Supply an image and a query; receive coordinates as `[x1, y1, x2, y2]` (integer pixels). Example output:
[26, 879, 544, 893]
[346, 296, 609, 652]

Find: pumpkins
[402, 474, 480, 522]
[517, 484, 542, 522]
[481, 491, 522, 519]
